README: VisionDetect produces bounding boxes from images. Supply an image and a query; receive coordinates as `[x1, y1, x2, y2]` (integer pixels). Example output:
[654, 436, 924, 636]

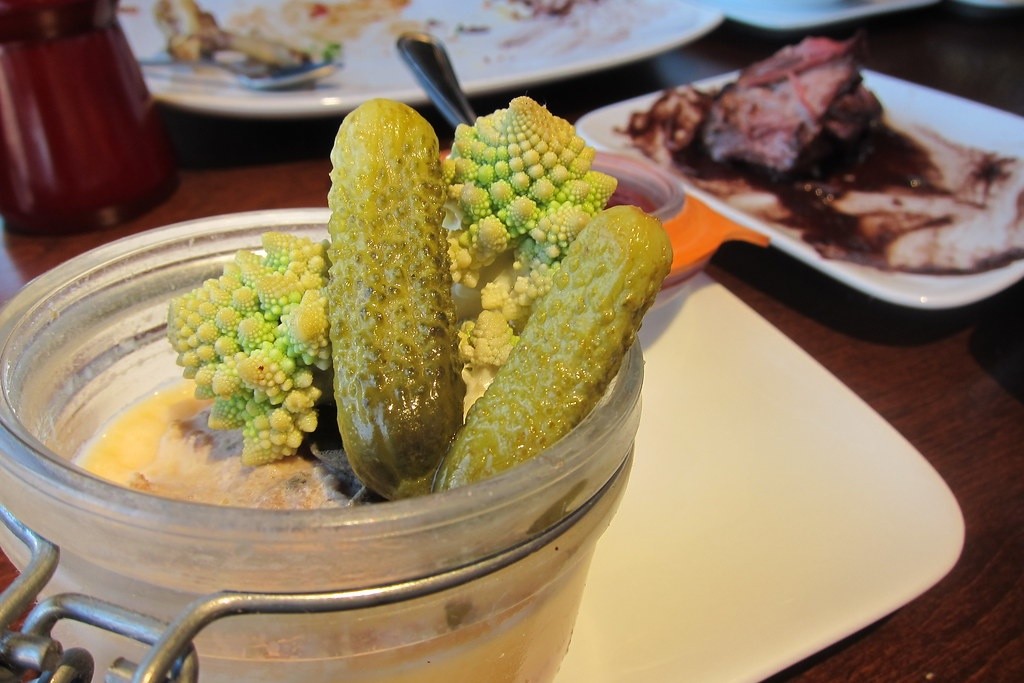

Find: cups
[0, 0, 175, 235]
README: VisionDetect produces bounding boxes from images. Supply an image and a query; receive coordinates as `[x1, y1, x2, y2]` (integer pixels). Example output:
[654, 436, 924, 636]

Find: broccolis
[161, 95, 618, 472]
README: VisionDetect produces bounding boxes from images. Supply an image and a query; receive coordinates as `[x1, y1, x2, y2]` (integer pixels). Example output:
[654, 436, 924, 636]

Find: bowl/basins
[0, 207, 645, 683]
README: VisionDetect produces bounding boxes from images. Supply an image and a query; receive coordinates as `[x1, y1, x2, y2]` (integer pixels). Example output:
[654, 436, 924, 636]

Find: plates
[576, 66, 1024, 308]
[117, 0, 725, 115]
[555, 272, 966, 683]
[725, 1, 948, 32]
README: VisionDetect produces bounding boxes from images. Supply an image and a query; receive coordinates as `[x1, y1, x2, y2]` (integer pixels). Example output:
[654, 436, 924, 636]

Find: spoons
[137, 60, 334, 90]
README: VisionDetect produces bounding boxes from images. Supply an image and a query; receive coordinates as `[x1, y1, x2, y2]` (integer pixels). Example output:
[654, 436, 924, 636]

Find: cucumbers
[425, 204, 673, 497]
[328, 98, 470, 501]
